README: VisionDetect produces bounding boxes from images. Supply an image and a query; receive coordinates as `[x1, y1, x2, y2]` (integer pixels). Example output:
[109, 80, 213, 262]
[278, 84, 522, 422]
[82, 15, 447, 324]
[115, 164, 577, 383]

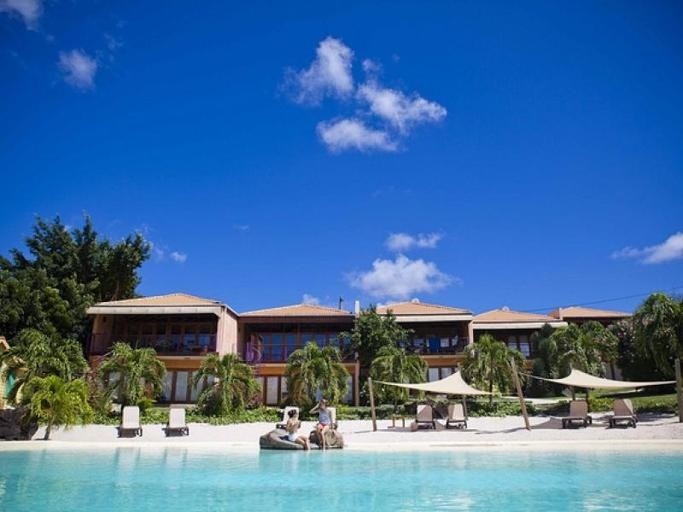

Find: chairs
[116, 403, 191, 439]
[554, 398, 639, 429]
[276, 402, 336, 430]
[409, 401, 466, 435]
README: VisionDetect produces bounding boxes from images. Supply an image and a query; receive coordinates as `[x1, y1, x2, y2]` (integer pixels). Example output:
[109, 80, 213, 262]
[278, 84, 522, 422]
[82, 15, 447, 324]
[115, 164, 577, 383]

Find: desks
[391, 414, 406, 429]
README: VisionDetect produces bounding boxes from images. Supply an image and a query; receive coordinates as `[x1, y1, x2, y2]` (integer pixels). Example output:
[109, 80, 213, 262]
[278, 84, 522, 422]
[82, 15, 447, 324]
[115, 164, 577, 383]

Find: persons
[308, 398, 332, 451]
[283, 409, 311, 450]
